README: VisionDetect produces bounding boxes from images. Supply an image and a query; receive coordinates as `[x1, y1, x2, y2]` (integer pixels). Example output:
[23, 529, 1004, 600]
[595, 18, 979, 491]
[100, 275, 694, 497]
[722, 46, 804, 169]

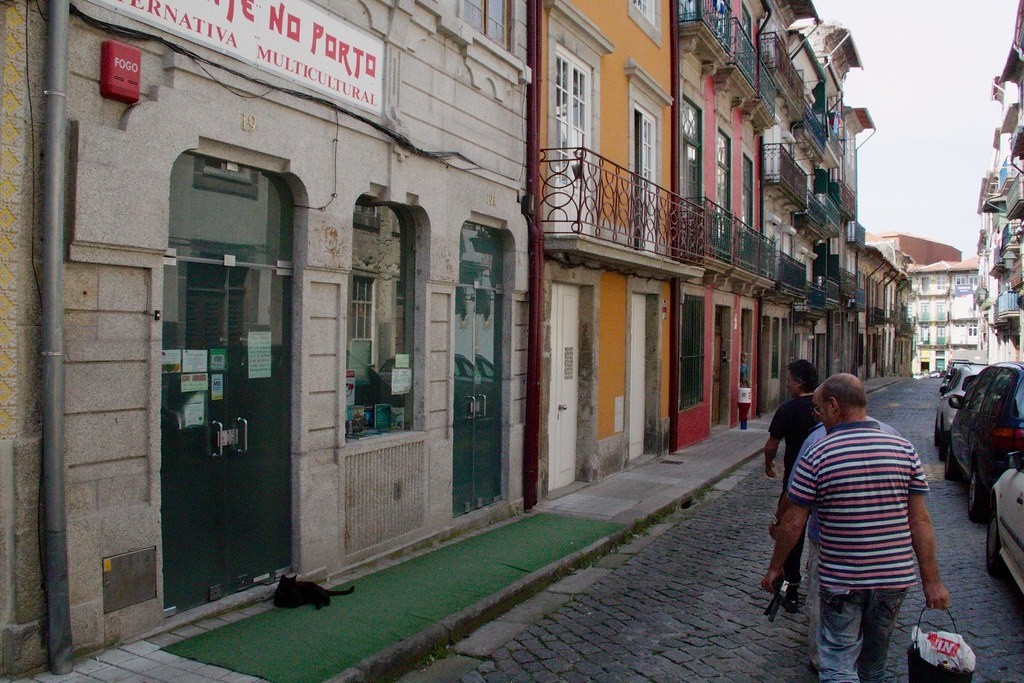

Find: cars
[945, 358, 977, 385]
[986, 449, 1024, 593]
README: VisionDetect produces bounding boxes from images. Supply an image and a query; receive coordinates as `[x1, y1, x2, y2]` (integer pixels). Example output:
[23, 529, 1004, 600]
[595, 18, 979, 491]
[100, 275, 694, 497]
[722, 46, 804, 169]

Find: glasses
[809, 405, 824, 414]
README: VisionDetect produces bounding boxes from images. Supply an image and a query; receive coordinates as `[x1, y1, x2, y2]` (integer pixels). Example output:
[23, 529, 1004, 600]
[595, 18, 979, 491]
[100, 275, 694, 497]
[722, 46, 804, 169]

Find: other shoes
[784, 597, 799, 613]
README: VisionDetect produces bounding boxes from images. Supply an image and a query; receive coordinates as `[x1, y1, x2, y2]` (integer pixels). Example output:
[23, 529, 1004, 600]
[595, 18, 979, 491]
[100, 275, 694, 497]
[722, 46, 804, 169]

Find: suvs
[943, 361, 1024, 524]
[934, 364, 985, 462]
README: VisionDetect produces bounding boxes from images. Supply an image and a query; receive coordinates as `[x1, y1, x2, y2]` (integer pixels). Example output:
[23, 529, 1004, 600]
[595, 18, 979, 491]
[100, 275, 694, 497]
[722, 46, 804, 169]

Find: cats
[273, 574, 355, 611]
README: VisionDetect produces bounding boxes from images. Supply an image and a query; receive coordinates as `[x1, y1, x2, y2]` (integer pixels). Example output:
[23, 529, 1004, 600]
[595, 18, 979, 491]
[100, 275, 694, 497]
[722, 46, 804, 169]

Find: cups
[346, 405, 375, 437]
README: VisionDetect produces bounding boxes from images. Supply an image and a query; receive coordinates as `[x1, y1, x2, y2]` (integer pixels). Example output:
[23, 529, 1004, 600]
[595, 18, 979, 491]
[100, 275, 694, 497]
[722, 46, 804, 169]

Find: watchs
[771, 515, 780, 527]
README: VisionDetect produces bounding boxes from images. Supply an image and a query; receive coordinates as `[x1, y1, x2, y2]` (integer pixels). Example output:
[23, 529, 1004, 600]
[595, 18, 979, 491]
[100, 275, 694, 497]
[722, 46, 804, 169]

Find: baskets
[907, 605, 974, 683]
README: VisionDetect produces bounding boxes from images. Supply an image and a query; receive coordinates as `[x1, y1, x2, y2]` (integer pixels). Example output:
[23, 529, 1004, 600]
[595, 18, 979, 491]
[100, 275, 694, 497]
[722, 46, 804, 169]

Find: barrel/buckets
[907, 604, 974, 683]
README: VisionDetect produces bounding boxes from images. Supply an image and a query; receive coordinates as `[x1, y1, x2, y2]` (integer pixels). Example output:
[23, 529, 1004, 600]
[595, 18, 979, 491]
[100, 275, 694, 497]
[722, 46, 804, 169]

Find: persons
[762, 372, 951, 683]
[740, 351, 748, 388]
[763, 359, 820, 613]
